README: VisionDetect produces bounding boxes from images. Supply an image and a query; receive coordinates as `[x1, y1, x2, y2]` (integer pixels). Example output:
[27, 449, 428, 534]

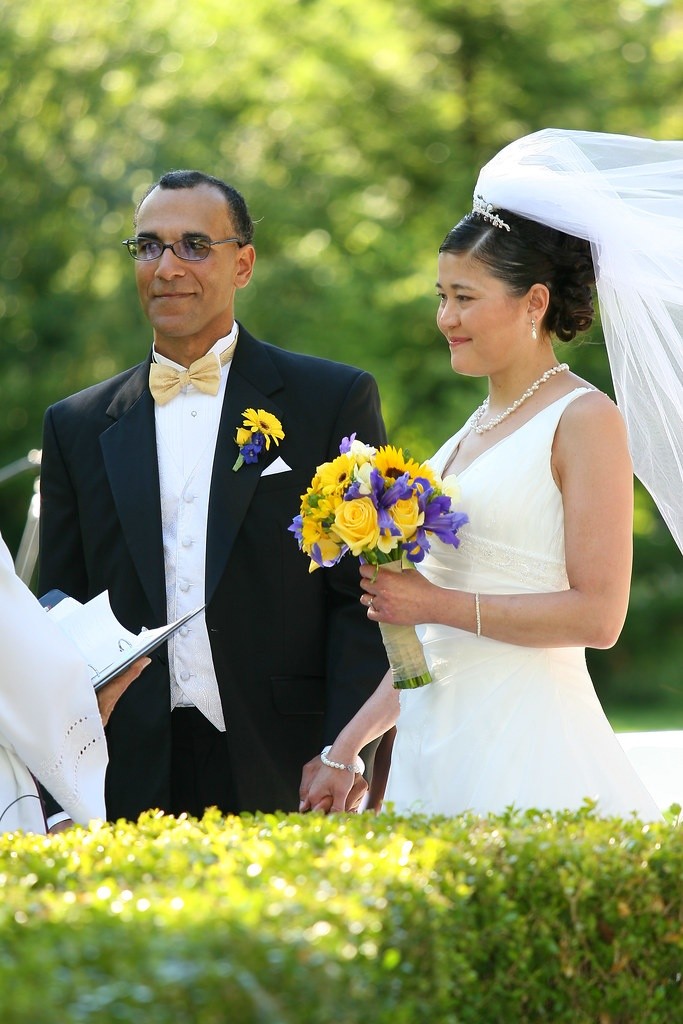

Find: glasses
[122, 236, 239, 261]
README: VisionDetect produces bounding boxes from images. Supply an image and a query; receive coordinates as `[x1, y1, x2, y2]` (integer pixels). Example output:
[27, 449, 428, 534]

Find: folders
[34, 585, 207, 694]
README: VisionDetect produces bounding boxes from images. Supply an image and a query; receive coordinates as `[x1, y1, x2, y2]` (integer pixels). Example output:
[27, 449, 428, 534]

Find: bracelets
[319, 744, 366, 774]
[474, 592, 482, 635]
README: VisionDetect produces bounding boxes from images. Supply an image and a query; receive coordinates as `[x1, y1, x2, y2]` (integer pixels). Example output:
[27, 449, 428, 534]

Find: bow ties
[149, 333, 237, 406]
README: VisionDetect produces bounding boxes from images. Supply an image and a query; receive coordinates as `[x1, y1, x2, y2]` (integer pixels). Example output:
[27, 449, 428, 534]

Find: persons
[39, 172, 384, 832]
[300, 127, 683, 816]
[3, 549, 155, 834]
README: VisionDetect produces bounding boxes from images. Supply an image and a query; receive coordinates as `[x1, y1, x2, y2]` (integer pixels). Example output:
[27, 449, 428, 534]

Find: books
[39, 588, 209, 694]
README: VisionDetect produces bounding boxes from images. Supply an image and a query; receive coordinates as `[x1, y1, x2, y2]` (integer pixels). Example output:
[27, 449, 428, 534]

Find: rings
[367, 596, 378, 610]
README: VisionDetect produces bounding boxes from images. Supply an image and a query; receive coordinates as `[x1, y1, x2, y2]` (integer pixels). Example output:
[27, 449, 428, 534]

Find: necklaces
[470, 363, 576, 436]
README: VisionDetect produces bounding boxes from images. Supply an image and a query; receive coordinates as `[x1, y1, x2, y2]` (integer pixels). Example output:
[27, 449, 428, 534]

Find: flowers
[288, 432, 470, 689]
[232, 408, 285, 471]
